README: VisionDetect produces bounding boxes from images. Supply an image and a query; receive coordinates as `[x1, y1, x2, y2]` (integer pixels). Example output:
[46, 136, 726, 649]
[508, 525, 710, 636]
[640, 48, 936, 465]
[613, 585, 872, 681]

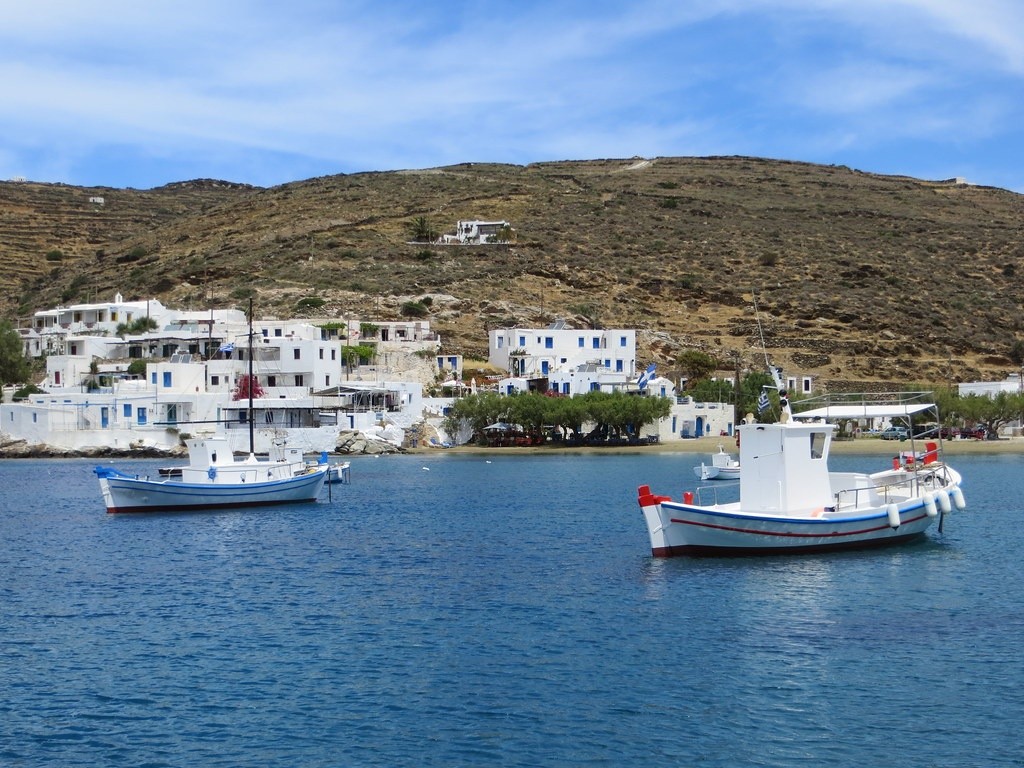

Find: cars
[961, 425, 985, 439]
[931, 425, 960, 438]
[881, 427, 907, 440]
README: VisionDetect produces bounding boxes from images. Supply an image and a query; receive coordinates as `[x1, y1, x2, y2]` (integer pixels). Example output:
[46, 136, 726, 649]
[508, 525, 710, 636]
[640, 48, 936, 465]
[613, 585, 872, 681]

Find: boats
[635, 387, 964, 561]
[694, 449, 741, 480]
[157, 465, 189, 476]
[322, 464, 352, 485]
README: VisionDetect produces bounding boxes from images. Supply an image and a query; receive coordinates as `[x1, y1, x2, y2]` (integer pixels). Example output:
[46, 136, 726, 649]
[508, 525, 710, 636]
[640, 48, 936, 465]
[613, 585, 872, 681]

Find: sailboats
[94, 297, 329, 514]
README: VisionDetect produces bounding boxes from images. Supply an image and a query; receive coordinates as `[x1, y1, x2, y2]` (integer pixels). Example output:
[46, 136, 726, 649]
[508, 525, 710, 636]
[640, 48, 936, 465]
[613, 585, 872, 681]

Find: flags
[757, 390, 770, 415]
[220, 342, 234, 351]
[636, 363, 655, 390]
